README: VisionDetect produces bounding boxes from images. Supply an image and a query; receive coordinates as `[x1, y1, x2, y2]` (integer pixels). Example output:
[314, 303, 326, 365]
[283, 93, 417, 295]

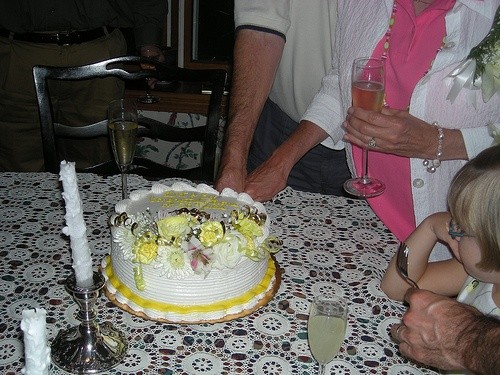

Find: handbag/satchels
[134, 109, 227, 182]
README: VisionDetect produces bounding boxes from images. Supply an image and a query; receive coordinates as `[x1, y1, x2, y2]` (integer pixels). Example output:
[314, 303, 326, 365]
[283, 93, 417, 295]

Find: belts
[0, 23, 116, 48]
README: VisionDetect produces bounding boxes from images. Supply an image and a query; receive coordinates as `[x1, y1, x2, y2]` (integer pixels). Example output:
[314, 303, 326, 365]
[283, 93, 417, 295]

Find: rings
[368, 137, 376, 147]
[396, 325, 402, 340]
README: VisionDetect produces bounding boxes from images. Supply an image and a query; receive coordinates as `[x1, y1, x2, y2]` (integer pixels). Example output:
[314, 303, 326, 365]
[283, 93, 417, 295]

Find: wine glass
[308, 294, 347, 375]
[343, 57, 386, 197]
[108, 99, 138, 200]
[136, 23, 162, 104]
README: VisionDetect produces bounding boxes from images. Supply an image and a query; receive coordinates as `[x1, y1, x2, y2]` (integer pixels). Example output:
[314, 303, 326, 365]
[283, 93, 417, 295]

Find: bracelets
[423, 121, 445, 173]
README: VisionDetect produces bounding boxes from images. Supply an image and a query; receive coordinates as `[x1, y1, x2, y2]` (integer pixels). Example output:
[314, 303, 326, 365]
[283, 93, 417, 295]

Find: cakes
[100, 182, 275, 322]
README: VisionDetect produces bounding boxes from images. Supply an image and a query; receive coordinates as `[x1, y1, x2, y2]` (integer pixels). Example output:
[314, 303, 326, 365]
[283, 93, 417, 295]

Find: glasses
[448, 218, 481, 239]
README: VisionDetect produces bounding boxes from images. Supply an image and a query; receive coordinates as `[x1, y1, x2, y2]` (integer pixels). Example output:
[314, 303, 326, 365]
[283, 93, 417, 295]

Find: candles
[58, 160, 95, 288]
[18, 305, 51, 375]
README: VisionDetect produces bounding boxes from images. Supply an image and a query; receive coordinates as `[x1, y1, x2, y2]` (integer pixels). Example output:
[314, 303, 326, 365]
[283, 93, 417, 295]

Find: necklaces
[381, 0, 456, 111]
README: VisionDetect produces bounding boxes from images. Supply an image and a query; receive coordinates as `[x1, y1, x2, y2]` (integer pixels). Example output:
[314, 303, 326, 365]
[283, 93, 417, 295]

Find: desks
[0, 171, 456, 375]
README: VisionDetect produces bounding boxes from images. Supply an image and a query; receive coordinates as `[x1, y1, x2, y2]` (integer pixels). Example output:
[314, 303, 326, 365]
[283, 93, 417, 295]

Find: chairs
[32, 55, 227, 191]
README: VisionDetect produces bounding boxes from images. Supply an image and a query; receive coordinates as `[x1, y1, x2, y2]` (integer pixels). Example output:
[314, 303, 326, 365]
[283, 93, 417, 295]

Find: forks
[396, 241, 420, 290]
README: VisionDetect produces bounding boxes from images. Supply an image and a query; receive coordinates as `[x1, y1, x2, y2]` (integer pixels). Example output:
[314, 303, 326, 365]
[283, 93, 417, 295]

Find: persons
[0, 0, 168, 173]
[212, 0, 500, 263]
[380, 145, 500, 375]
